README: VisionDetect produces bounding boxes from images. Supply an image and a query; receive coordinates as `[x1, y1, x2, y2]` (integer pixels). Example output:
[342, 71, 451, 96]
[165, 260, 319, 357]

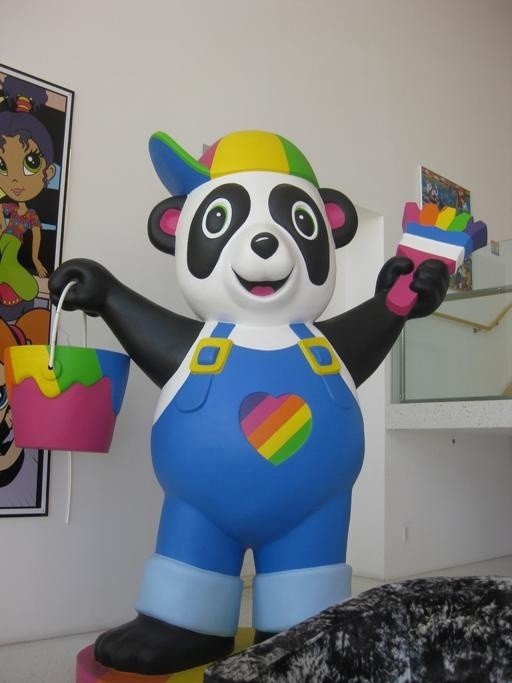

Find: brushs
[385, 201, 487, 316]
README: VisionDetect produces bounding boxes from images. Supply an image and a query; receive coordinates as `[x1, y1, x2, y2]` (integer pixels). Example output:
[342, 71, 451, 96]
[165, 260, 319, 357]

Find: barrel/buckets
[5, 276, 130, 454]
